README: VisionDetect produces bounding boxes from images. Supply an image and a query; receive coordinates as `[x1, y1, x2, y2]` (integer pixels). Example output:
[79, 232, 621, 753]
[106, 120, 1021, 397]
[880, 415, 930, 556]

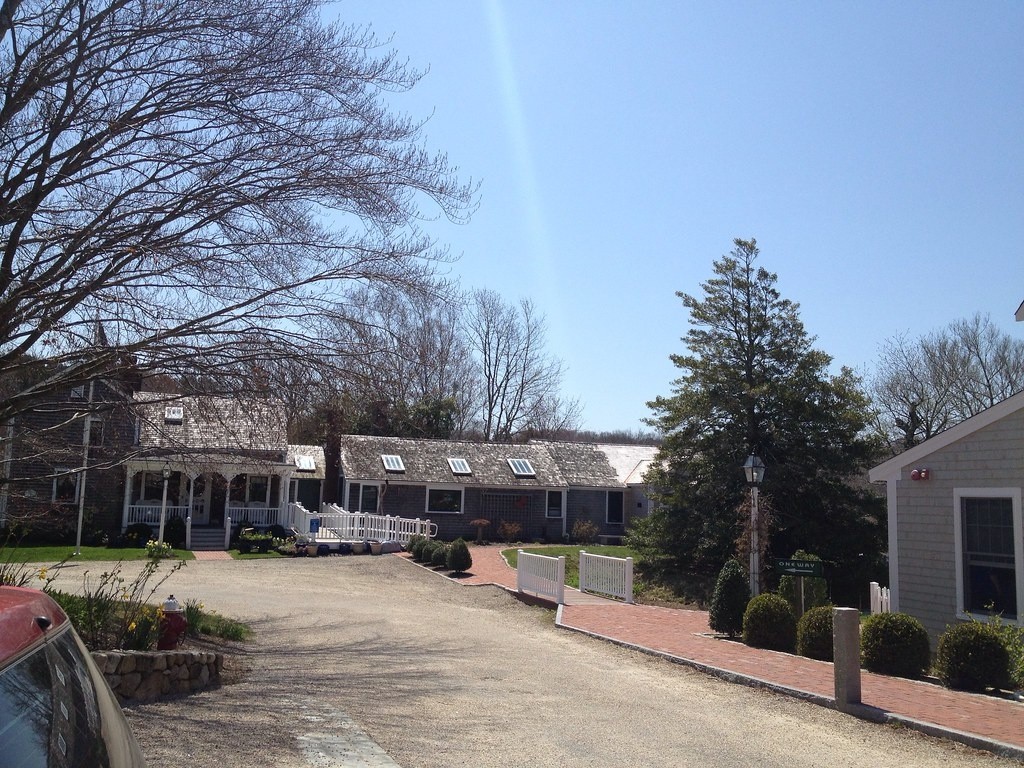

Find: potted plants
[294, 532, 306, 550]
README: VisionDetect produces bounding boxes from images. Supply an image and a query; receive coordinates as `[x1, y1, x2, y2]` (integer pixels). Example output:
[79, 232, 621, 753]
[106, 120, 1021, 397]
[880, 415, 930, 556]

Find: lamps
[155, 595, 185, 650]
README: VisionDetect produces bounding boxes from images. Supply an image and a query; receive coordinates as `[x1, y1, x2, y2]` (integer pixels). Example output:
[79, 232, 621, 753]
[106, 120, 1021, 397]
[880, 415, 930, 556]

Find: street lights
[741, 451, 767, 600]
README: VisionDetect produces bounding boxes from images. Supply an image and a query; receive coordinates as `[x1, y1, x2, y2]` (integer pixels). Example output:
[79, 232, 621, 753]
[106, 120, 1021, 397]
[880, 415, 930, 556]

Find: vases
[307, 541, 319, 556]
[339, 544, 351, 557]
[352, 541, 365, 555]
[370, 540, 382, 554]
[318, 544, 331, 557]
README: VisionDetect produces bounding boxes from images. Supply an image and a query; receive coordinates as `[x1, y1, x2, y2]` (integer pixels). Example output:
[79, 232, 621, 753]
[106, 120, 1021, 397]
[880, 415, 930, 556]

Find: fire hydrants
[157, 594, 189, 651]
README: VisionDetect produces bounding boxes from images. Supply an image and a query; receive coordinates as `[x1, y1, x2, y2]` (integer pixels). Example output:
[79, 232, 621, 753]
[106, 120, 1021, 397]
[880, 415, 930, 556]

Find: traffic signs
[774, 558, 825, 578]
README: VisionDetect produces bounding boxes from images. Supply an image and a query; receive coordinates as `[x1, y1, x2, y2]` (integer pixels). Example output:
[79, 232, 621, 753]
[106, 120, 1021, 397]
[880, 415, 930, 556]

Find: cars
[0, 582, 151, 768]
